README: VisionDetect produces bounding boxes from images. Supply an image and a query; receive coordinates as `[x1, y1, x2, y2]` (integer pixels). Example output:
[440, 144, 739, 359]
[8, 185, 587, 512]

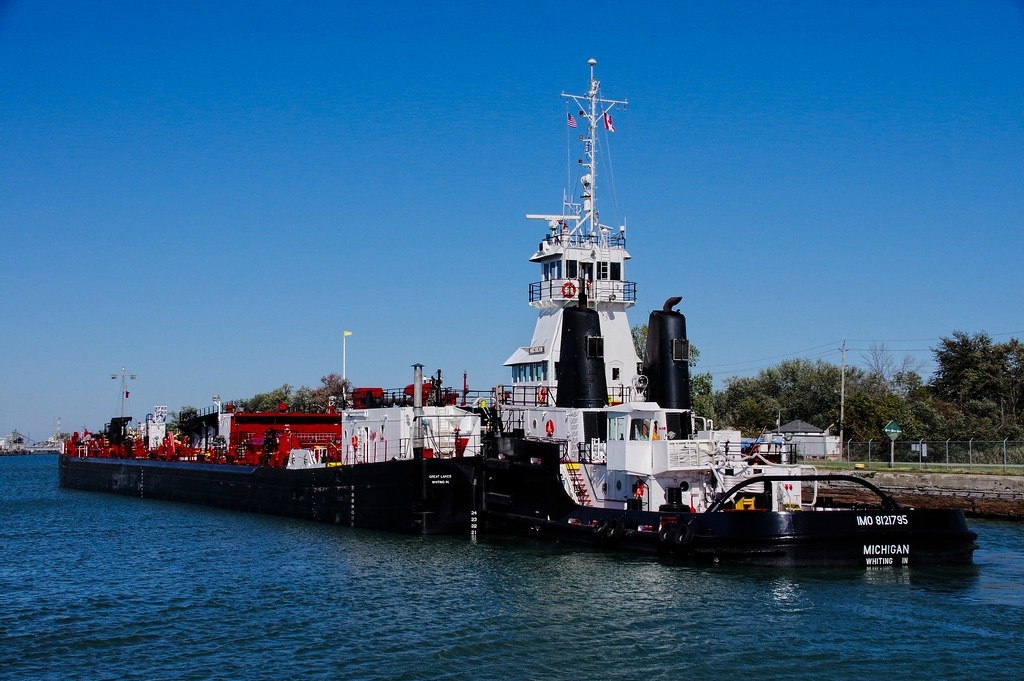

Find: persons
[86, 432, 91, 440]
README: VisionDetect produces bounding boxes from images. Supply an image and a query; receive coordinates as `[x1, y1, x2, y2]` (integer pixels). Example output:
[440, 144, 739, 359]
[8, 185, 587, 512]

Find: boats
[57, 56, 981, 575]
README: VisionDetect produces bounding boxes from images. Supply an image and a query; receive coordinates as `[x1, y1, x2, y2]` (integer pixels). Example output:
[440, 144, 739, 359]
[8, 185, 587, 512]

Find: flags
[604, 112, 616, 133]
[344, 331, 353, 336]
[568, 113, 577, 128]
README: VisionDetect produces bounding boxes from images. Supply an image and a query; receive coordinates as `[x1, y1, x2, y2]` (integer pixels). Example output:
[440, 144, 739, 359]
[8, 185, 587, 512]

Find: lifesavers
[538, 390, 545, 404]
[545, 420, 555, 436]
[352, 436, 358, 447]
[562, 282, 577, 298]
[634, 480, 644, 496]
